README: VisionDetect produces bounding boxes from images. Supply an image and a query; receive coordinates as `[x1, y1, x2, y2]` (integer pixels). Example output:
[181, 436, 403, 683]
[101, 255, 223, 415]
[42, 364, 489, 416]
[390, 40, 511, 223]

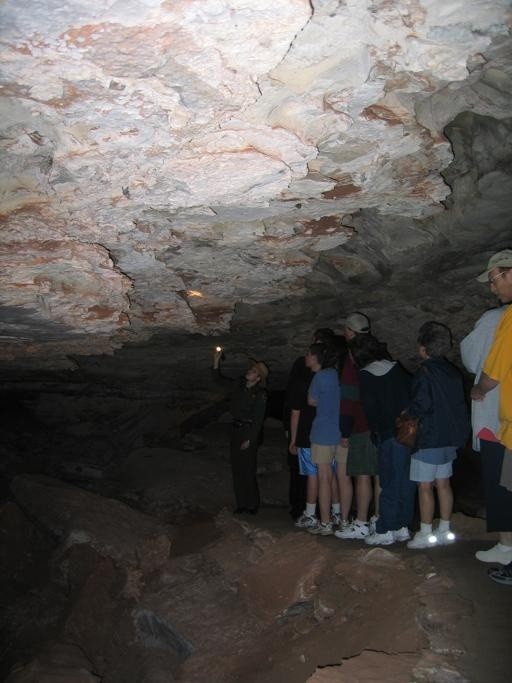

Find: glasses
[489, 270, 506, 282]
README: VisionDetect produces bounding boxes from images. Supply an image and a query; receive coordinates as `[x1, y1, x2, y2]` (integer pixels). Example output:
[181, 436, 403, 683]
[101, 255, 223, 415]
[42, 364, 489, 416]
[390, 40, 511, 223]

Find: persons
[474, 249, 512, 585]
[459, 304, 511, 565]
[349, 333, 413, 547]
[305, 343, 354, 537]
[406, 321, 468, 549]
[211, 345, 269, 515]
[333, 310, 383, 541]
[286, 328, 344, 528]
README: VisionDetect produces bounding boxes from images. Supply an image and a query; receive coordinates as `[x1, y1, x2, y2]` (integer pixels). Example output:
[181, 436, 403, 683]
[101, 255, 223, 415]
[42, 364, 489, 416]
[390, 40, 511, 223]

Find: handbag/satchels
[394, 410, 421, 446]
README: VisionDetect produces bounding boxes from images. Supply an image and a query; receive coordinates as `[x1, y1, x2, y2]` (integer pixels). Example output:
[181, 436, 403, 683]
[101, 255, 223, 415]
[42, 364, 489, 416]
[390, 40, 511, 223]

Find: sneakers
[475, 545, 512, 584]
[295, 510, 456, 549]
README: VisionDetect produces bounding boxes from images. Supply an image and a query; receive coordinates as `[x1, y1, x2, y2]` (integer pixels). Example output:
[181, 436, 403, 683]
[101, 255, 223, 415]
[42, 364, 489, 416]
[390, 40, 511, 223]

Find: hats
[338, 313, 371, 333]
[248, 357, 268, 387]
[476, 249, 512, 282]
